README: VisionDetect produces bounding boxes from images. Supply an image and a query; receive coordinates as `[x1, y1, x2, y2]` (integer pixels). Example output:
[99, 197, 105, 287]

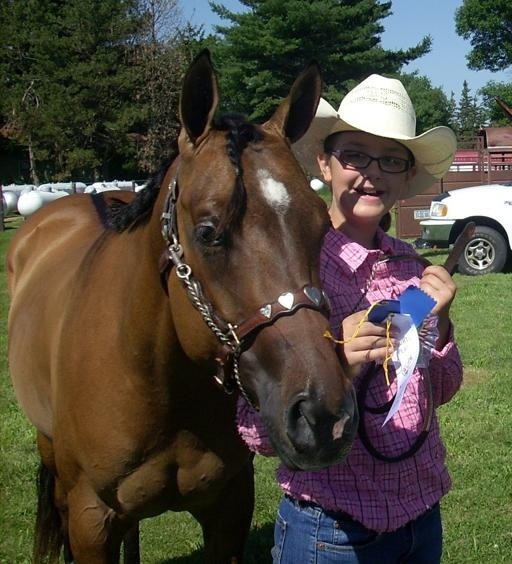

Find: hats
[290, 73, 457, 200]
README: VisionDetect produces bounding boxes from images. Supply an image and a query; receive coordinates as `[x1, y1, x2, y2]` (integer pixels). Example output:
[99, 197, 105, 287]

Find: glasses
[326, 146, 411, 174]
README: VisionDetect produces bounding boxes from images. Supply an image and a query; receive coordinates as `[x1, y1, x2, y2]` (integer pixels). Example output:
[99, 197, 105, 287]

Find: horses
[6, 45, 360, 564]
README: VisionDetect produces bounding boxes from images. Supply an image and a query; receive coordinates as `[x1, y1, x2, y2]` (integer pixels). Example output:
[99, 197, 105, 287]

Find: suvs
[419, 182, 512, 274]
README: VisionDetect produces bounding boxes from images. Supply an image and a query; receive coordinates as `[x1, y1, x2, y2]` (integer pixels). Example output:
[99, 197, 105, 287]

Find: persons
[236, 74, 465, 564]
